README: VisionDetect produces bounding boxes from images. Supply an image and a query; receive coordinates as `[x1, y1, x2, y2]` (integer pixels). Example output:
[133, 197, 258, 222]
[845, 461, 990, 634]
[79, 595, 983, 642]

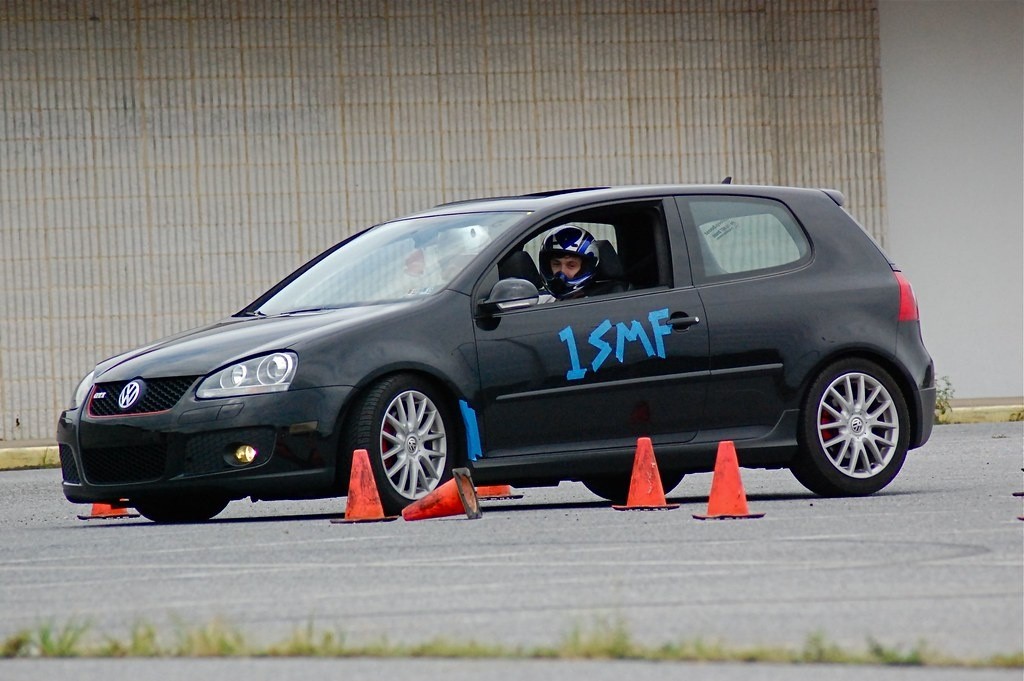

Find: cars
[56, 178, 939, 525]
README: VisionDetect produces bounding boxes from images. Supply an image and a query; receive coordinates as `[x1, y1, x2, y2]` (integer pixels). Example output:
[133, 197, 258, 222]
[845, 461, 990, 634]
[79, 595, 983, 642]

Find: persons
[539, 225, 601, 304]
[434, 229, 466, 280]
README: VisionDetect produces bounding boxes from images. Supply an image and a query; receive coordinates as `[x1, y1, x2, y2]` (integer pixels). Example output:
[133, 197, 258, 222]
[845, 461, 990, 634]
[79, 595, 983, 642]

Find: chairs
[497, 252, 544, 291]
[595, 240, 620, 281]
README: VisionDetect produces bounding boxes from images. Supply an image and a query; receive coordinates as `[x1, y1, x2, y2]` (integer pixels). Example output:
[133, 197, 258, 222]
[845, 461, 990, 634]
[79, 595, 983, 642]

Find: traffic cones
[403, 467, 483, 522]
[611, 436, 682, 511]
[76, 497, 141, 520]
[330, 449, 400, 524]
[474, 484, 527, 500]
[691, 440, 767, 520]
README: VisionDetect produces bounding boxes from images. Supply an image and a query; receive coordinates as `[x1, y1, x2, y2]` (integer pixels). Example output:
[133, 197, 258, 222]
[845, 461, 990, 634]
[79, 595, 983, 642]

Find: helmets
[539, 225, 600, 299]
[439, 224, 493, 270]
